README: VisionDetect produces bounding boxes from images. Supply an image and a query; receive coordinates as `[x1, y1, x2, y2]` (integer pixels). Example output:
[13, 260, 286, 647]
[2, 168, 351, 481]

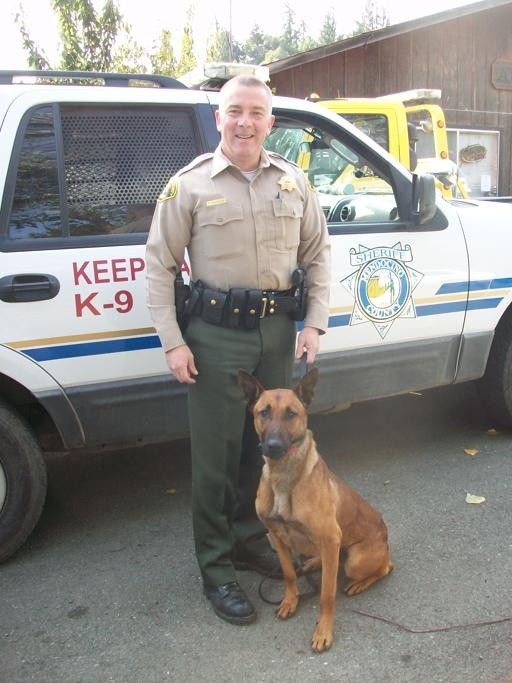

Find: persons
[144, 71, 336, 626]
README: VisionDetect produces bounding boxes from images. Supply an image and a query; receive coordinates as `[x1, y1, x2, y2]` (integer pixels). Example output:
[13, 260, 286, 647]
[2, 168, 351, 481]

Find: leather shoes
[230, 535, 300, 580]
[203, 579, 257, 625]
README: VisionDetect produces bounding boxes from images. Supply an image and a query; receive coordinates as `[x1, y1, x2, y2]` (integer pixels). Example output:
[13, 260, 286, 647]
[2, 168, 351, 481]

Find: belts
[260, 287, 296, 318]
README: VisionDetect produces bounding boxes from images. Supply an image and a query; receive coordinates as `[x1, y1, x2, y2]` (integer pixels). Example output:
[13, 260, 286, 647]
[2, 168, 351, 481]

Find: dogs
[237, 368, 393, 652]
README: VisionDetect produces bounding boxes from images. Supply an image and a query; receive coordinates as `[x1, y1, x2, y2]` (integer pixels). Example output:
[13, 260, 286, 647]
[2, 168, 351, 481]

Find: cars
[0, 68, 510, 565]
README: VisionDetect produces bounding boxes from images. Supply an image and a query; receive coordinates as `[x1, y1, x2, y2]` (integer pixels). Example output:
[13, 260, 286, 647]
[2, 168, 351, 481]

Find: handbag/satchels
[290, 268, 308, 321]
[175, 271, 260, 329]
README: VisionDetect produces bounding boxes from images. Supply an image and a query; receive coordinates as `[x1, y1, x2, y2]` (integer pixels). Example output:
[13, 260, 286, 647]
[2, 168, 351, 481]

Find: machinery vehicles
[299, 88, 467, 200]
